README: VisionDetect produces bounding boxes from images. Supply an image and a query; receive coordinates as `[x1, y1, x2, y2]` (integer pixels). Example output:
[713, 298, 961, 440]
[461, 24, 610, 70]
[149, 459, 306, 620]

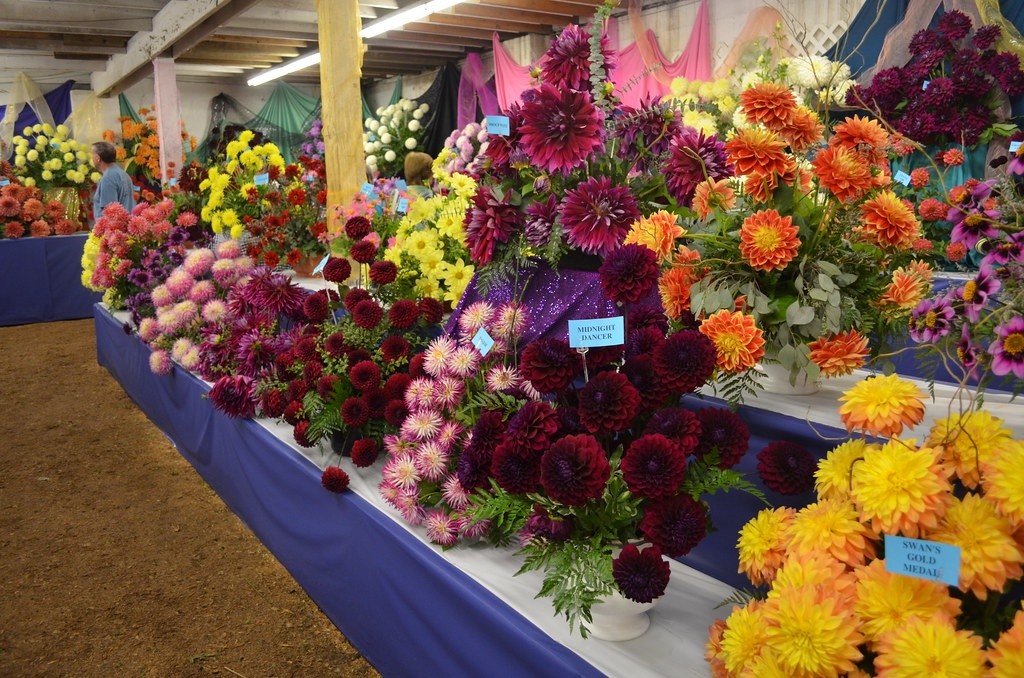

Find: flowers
[0, 0, 1024, 678]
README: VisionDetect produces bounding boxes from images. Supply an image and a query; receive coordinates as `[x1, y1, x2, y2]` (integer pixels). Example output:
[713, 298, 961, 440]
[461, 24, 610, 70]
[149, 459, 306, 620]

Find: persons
[404, 151, 435, 199]
[92, 141, 135, 222]
